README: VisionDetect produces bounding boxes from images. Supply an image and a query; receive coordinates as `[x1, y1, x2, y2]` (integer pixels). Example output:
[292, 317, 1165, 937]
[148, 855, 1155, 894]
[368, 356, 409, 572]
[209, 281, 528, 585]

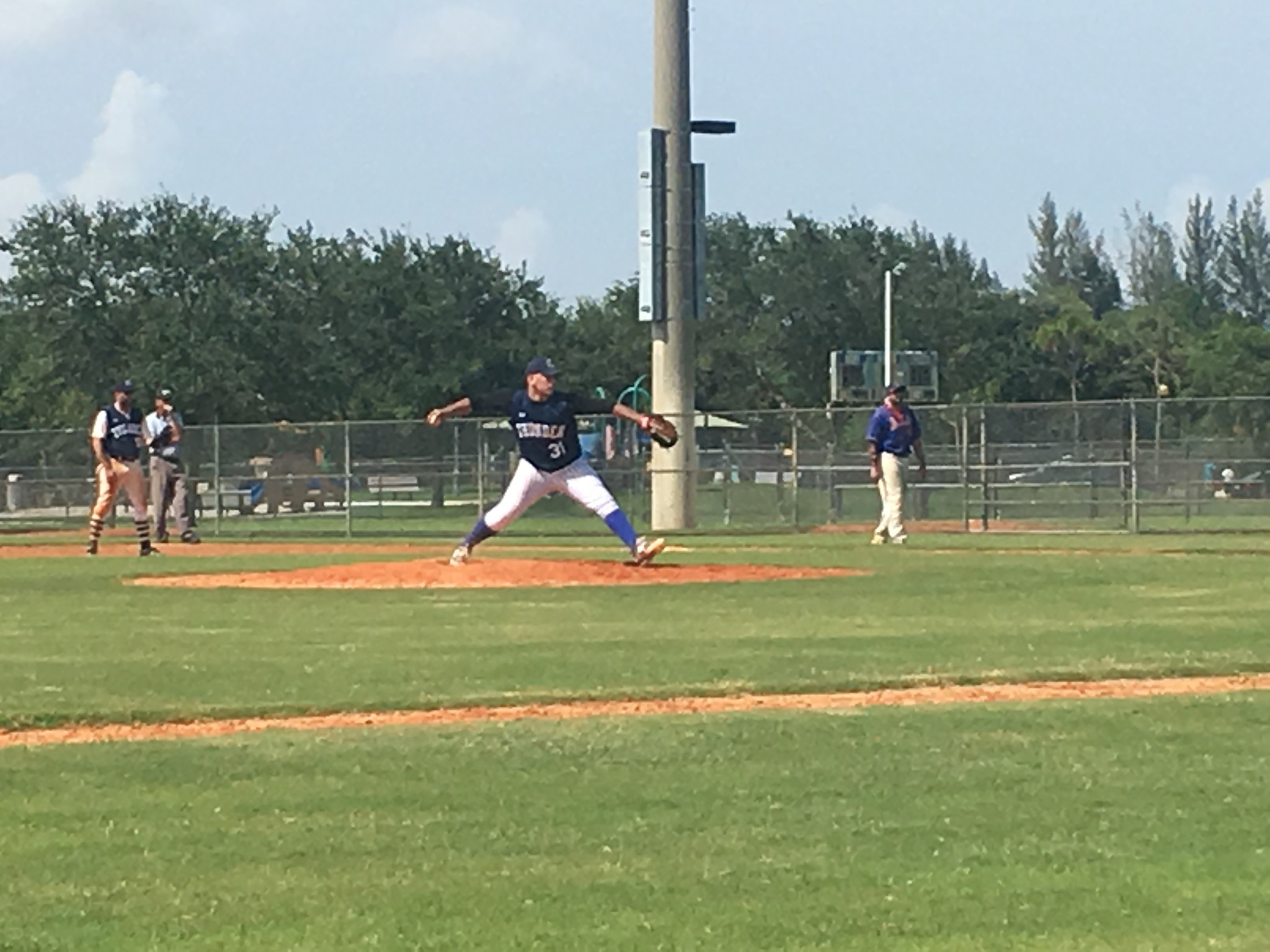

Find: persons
[423, 350, 680, 565]
[264, 418, 343, 512]
[84, 379, 161, 557]
[141, 387, 201, 544]
[861, 382, 927, 547]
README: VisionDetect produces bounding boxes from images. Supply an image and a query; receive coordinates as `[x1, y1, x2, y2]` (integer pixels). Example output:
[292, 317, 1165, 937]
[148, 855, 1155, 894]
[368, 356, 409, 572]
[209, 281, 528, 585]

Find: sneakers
[449, 542, 470, 567]
[634, 538, 664, 566]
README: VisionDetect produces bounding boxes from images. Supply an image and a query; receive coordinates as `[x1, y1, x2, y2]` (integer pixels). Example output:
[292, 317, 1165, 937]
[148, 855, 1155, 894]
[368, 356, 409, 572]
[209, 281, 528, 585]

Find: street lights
[883, 260, 907, 388]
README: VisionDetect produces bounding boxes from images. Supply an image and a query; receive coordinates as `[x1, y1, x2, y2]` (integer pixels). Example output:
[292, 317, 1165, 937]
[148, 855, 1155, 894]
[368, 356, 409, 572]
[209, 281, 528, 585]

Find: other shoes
[155, 534, 166, 542]
[185, 535, 201, 543]
[86, 547, 95, 555]
[140, 548, 160, 555]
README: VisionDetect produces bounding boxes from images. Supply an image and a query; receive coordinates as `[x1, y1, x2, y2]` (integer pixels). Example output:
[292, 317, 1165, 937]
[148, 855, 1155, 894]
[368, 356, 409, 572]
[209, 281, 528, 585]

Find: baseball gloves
[150, 425, 173, 449]
[646, 414, 679, 448]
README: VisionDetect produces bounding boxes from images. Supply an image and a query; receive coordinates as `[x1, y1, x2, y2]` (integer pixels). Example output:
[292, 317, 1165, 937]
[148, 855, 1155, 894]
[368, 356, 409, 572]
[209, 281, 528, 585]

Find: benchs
[193, 489, 252, 519]
[368, 475, 423, 501]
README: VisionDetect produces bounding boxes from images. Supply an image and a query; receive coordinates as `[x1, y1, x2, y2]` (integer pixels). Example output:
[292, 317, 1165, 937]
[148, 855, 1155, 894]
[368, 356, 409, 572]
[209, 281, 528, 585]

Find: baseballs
[429, 414, 442, 427]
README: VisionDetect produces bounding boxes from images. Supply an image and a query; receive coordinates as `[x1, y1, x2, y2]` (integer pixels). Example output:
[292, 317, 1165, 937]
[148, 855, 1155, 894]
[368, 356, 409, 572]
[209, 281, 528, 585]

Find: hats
[156, 389, 173, 402]
[117, 380, 136, 393]
[885, 384, 905, 396]
[527, 356, 561, 377]
